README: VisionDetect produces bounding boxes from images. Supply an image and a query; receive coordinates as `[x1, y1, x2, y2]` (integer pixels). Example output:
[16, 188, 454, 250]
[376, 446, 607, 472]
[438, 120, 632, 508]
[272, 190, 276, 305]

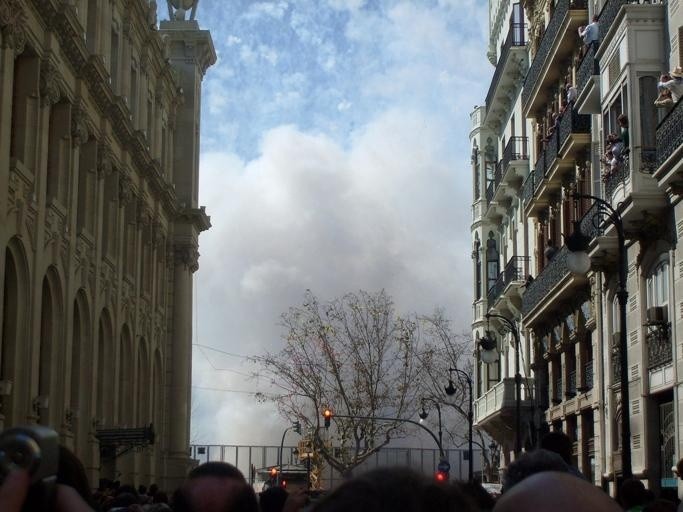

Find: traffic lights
[293, 421, 299, 433]
[436, 472, 449, 480]
[324, 408, 331, 427]
[271, 468, 277, 480]
[282, 480, 287, 486]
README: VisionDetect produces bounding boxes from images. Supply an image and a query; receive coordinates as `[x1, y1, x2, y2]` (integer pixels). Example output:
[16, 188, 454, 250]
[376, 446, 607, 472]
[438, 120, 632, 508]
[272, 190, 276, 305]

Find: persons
[1, 428, 683, 512]
[543, 238, 559, 262]
[538, 13, 683, 186]
[520, 275, 534, 289]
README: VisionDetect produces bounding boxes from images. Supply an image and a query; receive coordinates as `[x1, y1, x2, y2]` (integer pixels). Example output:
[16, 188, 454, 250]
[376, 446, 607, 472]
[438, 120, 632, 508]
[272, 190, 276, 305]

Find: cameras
[0, 422, 59, 512]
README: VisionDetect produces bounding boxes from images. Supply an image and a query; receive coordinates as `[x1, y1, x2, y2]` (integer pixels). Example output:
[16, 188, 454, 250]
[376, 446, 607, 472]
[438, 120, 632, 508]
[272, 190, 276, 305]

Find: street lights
[476, 313, 522, 461]
[444, 367, 496, 482]
[418, 397, 443, 462]
[559, 192, 632, 476]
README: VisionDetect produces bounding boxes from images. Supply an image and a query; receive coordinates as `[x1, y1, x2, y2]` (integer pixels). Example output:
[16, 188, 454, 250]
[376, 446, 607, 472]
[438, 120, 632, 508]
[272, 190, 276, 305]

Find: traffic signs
[437, 461, 450, 474]
